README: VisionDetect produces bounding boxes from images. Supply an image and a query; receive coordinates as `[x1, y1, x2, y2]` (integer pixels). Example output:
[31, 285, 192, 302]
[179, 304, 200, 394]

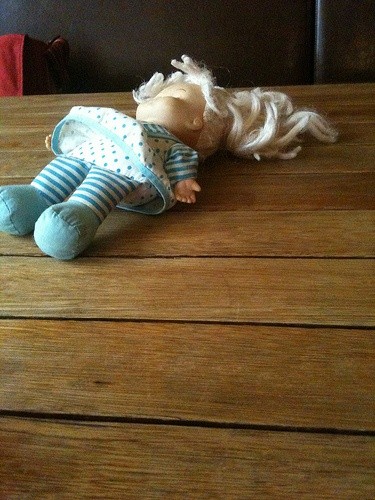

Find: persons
[0, 54, 339, 262]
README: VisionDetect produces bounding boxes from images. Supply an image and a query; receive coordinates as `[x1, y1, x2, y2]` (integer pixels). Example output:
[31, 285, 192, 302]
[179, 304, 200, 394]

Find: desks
[0, 82, 375, 500]
